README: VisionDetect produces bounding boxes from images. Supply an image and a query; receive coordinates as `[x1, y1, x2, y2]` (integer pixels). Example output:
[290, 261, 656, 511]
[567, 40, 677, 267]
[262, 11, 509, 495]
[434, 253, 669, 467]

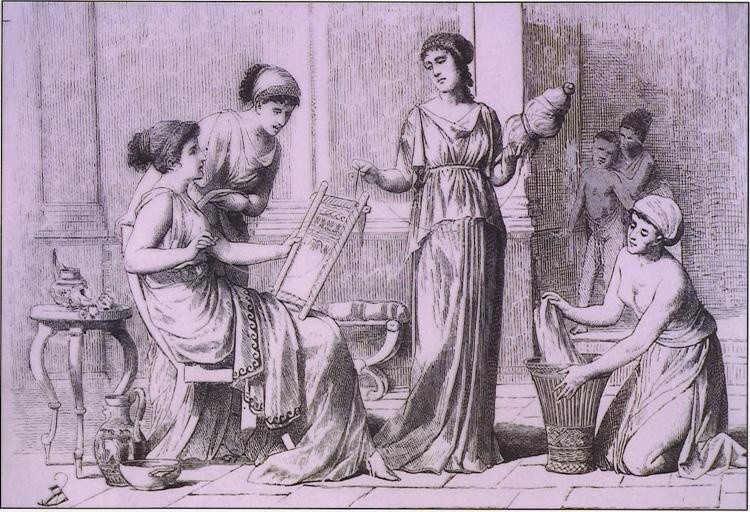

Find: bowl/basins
[119, 459, 181, 490]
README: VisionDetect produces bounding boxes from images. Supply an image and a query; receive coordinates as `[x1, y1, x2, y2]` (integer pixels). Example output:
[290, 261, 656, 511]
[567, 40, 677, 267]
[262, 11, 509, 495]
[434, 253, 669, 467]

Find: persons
[350, 34, 524, 473]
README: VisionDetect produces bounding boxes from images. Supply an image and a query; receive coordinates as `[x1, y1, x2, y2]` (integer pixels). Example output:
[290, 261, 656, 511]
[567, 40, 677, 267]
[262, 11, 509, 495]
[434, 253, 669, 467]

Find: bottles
[49, 267, 90, 309]
[94, 387, 147, 488]
